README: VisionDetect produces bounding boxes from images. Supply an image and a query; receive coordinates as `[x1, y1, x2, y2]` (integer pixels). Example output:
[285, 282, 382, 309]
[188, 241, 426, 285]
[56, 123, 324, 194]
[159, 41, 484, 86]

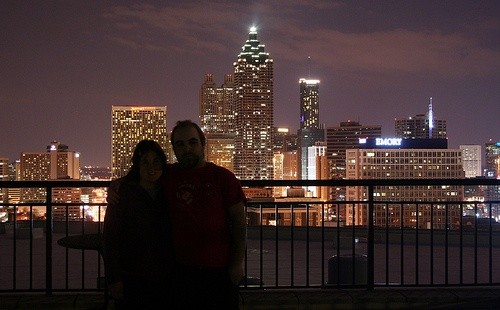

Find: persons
[105, 117, 246, 309]
[102, 139, 192, 309]
